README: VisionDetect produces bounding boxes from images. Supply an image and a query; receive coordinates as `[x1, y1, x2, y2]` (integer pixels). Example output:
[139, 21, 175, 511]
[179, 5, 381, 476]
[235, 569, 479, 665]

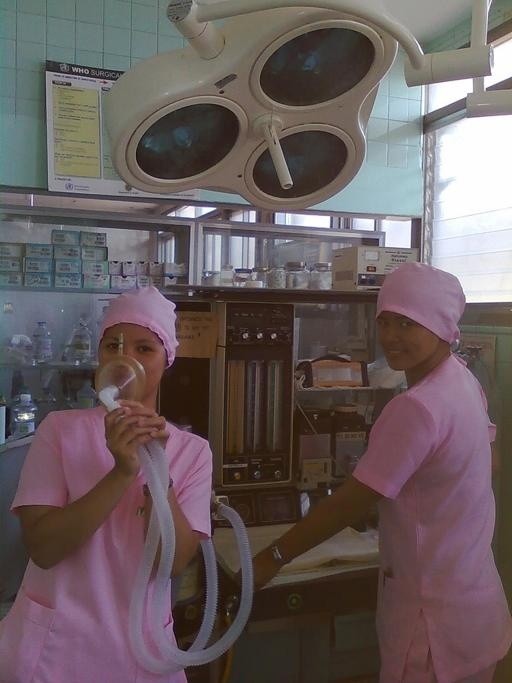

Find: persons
[233, 260, 512, 682]
[0, 283, 214, 683]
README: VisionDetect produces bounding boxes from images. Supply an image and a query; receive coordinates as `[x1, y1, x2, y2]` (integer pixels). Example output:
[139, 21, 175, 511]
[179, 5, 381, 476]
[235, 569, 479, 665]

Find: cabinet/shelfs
[0, 206, 195, 292]
[196, 222, 385, 292]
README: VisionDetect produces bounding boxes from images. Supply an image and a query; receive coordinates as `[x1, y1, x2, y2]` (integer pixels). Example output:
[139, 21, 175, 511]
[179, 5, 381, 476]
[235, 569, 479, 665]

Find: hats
[99, 284, 179, 370]
[373, 259, 466, 346]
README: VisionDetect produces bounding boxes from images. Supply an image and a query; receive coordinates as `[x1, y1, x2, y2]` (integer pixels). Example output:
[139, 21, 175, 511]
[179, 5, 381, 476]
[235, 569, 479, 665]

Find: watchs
[268, 539, 291, 570]
